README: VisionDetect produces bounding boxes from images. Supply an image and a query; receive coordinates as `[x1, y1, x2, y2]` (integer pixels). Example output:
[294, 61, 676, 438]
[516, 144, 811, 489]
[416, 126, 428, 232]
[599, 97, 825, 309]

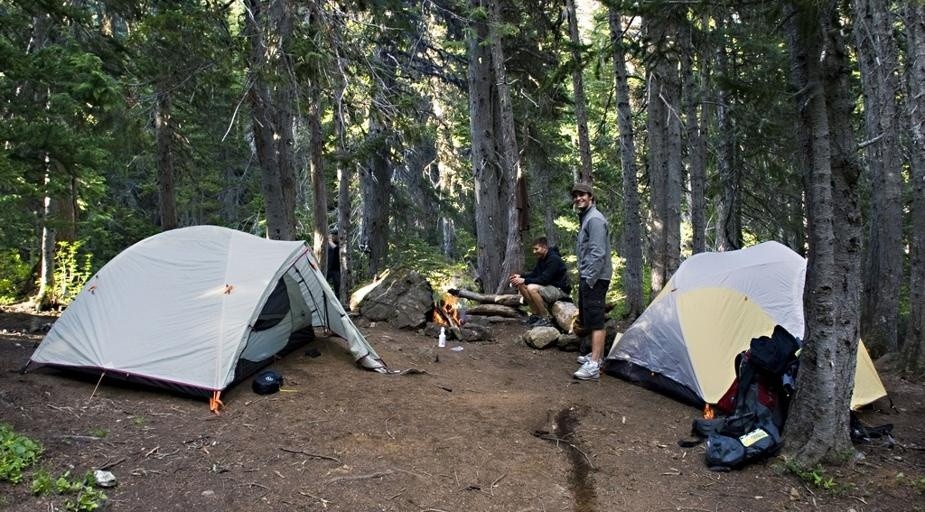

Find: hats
[572, 183, 592, 194]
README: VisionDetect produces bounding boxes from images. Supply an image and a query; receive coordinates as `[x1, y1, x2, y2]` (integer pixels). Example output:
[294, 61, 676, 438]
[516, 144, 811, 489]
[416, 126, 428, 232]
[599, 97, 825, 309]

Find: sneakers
[521, 315, 541, 326]
[576, 353, 605, 368]
[533, 316, 554, 327]
[574, 360, 600, 381]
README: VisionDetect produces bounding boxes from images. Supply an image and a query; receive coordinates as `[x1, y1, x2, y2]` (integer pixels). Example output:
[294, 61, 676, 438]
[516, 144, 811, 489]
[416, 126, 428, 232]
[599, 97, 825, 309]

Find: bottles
[438, 326, 446, 347]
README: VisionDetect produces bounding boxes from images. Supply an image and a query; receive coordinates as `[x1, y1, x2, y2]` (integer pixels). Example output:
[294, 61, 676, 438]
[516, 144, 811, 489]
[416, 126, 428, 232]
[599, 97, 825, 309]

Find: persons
[509, 237, 571, 328]
[571, 184, 613, 381]
[327, 231, 339, 300]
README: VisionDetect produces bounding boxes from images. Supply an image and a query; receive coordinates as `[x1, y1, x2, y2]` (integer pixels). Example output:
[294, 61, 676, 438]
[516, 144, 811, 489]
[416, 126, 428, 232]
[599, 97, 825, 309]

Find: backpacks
[678, 325, 800, 473]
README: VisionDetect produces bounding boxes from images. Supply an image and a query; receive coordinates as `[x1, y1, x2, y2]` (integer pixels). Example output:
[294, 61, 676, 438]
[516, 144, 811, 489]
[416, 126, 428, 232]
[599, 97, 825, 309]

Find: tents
[20, 224, 387, 410]
[606, 240, 888, 419]
